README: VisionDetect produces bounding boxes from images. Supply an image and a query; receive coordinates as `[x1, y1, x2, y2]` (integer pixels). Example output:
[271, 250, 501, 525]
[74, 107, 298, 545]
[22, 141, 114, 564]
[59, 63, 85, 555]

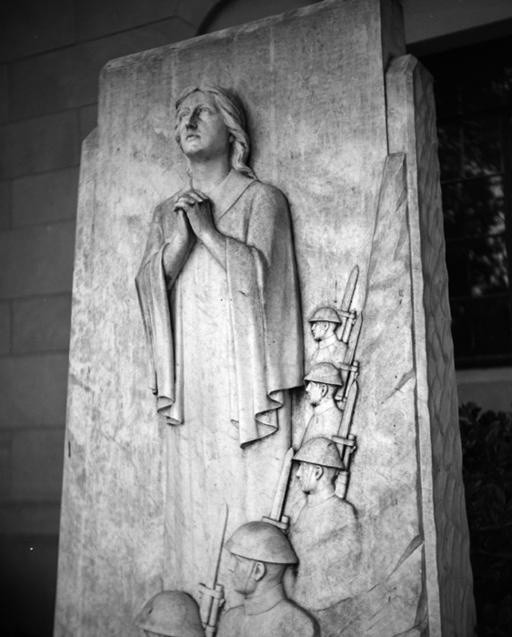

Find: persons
[215, 521, 321, 636]
[284, 434, 361, 605]
[134, 589, 207, 637]
[299, 360, 346, 446]
[133, 80, 307, 617]
[308, 306, 347, 364]
